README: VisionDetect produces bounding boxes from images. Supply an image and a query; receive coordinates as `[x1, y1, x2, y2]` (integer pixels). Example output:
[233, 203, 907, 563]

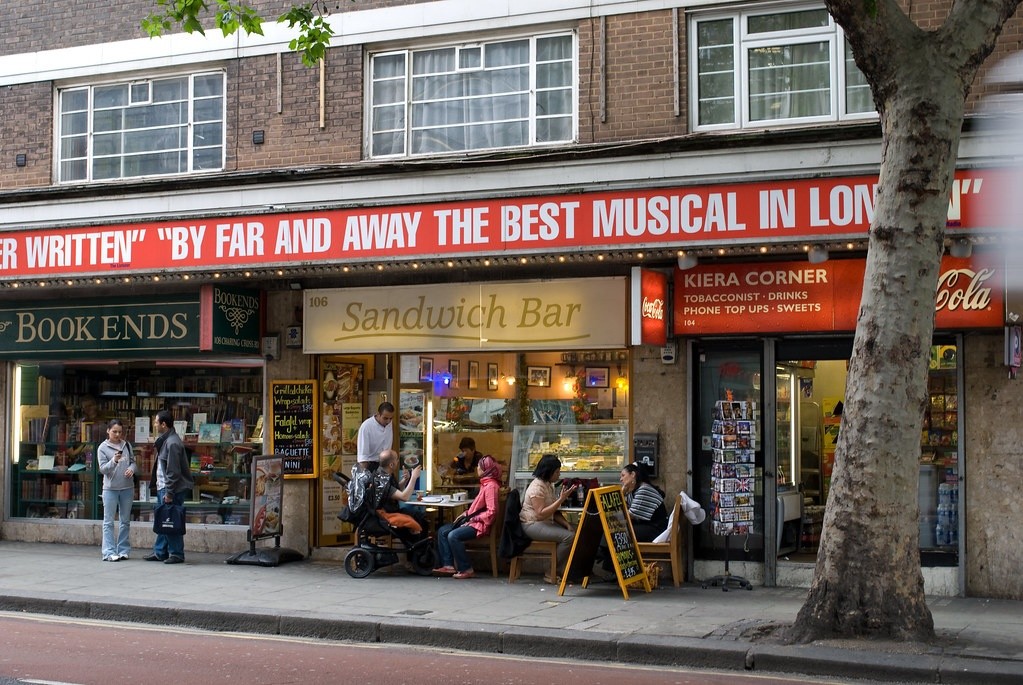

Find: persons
[369, 450, 429, 572]
[518, 454, 575, 585]
[356, 402, 394, 545]
[450, 438, 481, 510]
[430, 455, 500, 581]
[69, 397, 102, 470]
[143, 411, 194, 563]
[602, 460, 667, 584]
[97, 419, 137, 561]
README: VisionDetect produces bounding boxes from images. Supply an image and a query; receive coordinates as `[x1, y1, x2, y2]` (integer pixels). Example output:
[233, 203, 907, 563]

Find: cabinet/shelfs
[16, 368, 263, 526]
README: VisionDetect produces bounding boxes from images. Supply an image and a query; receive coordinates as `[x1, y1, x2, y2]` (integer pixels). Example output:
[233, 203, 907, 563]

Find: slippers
[544, 576, 561, 584]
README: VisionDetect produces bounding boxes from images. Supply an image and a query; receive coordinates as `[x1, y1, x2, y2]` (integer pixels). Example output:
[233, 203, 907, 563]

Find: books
[19, 372, 264, 526]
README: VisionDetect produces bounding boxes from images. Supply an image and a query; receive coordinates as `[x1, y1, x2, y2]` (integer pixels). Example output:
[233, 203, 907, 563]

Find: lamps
[501, 369, 515, 385]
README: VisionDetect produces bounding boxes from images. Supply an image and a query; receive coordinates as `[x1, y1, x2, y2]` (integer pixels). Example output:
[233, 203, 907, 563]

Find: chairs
[453, 495, 507, 578]
[507, 489, 559, 586]
[637, 495, 688, 588]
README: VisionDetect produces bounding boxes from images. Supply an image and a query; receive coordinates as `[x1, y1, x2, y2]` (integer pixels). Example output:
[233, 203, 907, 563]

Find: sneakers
[102, 555, 120, 561]
[432, 567, 457, 576]
[119, 554, 128, 560]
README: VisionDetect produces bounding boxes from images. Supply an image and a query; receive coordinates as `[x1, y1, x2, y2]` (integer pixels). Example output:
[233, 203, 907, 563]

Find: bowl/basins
[422, 497, 443, 503]
[404, 458, 419, 468]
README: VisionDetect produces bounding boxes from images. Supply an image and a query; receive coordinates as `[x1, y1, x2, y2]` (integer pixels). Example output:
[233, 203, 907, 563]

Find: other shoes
[144, 552, 160, 560]
[453, 569, 475, 578]
[164, 557, 183, 563]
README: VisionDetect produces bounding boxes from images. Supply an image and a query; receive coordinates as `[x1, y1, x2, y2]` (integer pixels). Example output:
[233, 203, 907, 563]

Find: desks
[405, 499, 474, 569]
[555, 505, 584, 524]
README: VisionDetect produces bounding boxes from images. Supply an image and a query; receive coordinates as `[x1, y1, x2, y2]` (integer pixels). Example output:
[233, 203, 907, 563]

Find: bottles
[577, 484, 584, 507]
[801, 505, 826, 548]
[936, 483, 958, 545]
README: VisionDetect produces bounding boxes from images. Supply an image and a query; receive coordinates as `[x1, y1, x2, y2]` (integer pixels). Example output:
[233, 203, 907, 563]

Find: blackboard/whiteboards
[269, 380, 319, 478]
[561, 483, 647, 584]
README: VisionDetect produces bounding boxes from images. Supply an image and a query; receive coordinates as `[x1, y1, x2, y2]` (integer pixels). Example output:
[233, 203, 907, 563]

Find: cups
[453, 492, 466, 501]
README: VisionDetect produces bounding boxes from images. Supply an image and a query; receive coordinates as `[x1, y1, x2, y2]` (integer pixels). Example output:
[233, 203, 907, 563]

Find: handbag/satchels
[560, 477, 598, 506]
[153, 502, 186, 535]
[626, 563, 660, 589]
[442, 516, 468, 536]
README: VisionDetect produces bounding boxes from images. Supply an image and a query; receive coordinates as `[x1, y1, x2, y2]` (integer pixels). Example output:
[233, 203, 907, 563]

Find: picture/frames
[468, 361, 479, 390]
[419, 357, 433, 381]
[488, 363, 499, 391]
[585, 366, 609, 388]
[526, 366, 551, 387]
[448, 360, 460, 389]
